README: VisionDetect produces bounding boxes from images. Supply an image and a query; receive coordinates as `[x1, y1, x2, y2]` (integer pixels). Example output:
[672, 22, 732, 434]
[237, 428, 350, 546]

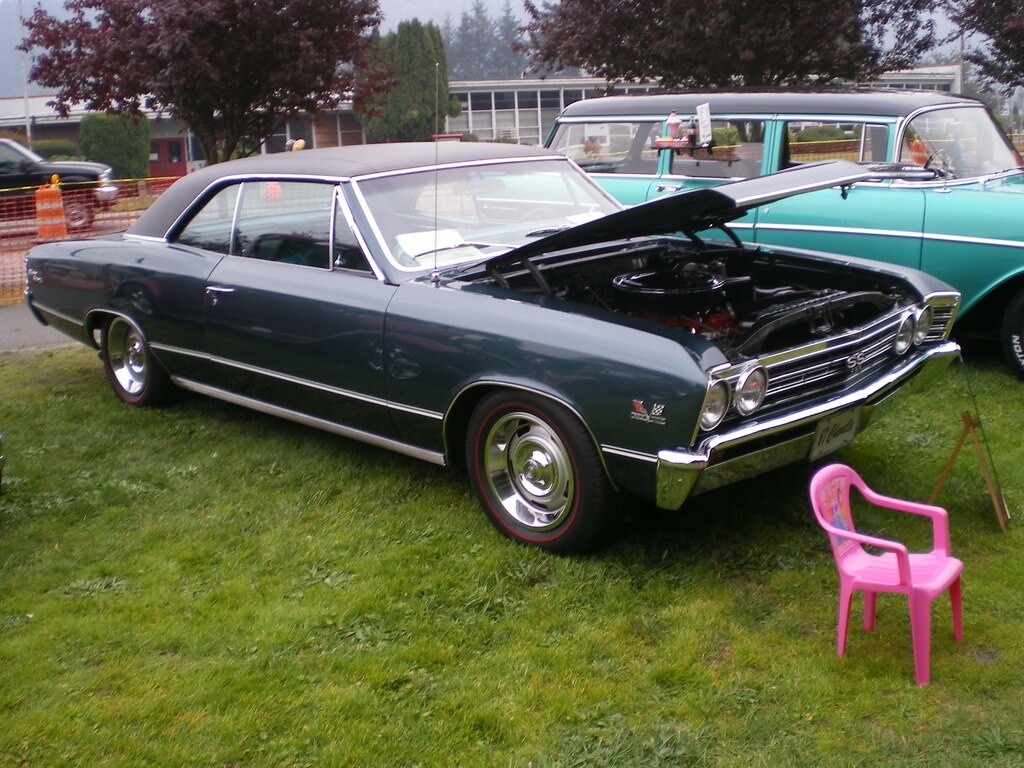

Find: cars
[470, 91, 1024, 381]
[0, 139, 119, 234]
[25, 142, 963, 558]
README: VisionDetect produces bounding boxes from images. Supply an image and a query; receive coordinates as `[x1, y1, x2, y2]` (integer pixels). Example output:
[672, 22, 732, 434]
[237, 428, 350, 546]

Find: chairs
[810, 463, 965, 685]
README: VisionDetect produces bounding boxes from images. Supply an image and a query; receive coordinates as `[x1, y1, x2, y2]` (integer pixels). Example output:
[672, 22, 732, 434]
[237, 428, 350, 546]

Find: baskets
[654, 139, 688, 147]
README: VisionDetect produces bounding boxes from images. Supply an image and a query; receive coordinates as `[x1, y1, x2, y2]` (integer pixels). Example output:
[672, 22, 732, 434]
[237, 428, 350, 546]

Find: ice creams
[666, 112, 682, 139]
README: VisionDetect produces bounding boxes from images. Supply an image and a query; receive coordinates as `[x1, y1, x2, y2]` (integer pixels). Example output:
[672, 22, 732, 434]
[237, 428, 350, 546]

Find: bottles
[686, 115, 697, 146]
[667, 110, 682, 138]
